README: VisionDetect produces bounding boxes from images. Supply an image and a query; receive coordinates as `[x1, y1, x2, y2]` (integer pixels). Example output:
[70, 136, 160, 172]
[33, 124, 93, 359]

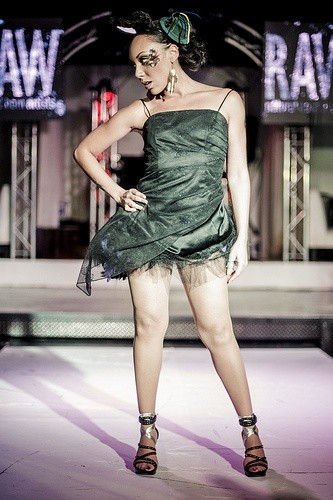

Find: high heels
[238, 414, 268, 477]
[133, 413, 159, 474]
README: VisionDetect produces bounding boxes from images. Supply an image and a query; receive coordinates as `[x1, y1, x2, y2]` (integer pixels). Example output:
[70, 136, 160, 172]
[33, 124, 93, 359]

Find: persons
[72, 10, 270, 478]
